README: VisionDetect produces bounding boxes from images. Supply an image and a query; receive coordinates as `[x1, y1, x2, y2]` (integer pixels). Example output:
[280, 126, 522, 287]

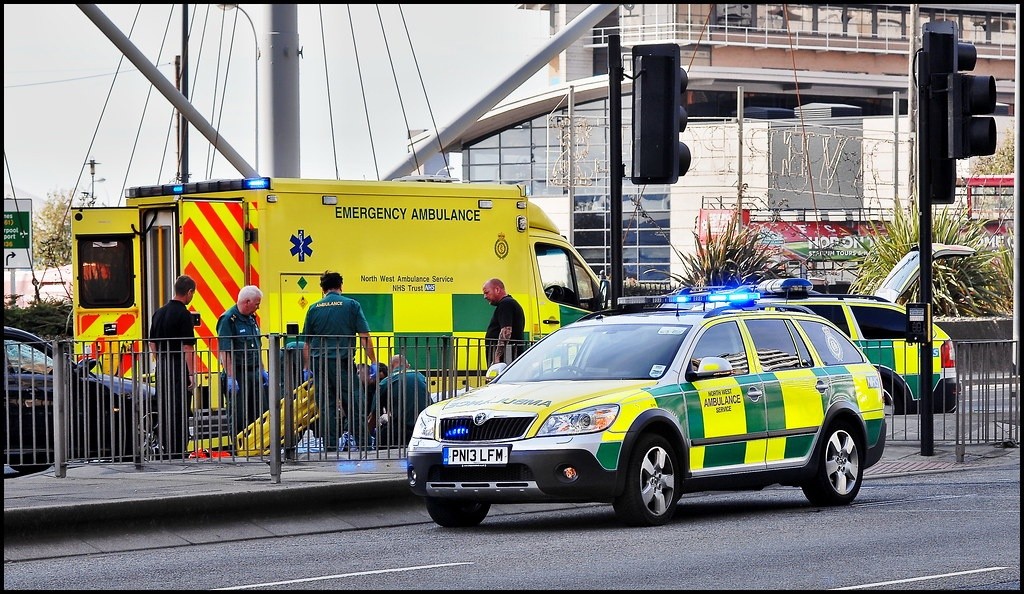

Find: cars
[5, 325, 157, 469]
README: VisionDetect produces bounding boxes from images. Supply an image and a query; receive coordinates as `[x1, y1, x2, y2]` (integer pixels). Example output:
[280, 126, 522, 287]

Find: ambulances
[408, 293, 887, 525]
[724, 243, 975, 416]
[70, 175, 612, 451]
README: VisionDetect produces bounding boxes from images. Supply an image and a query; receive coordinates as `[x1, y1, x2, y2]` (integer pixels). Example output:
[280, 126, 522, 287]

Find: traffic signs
[5, 198, 32, 271]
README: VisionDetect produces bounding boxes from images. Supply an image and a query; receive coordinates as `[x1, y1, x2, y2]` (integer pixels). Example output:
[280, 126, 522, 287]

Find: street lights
[218, 3, 260, 171]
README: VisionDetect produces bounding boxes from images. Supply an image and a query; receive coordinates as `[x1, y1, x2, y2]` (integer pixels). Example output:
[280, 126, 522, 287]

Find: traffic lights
[922, 22, 997, 205]
[631, 43, 691, 186]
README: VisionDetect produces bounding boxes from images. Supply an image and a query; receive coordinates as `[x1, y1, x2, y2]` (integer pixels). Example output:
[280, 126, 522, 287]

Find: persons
[482, 277, 526, 386]
[149, 274, 196, 461]
[218, 269, 434, 458]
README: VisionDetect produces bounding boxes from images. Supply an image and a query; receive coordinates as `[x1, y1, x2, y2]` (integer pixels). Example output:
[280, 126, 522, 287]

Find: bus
[700, 176, 1015, 264]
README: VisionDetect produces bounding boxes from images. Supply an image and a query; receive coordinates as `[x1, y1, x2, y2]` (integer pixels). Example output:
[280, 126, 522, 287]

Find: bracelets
[189, 375, 194, 376]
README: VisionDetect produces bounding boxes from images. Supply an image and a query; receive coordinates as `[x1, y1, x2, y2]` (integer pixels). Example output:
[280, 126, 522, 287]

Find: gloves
[303, 369, 313, 381]
[369, 363, 379, 379]
[261, 369, 269, 387]
[228, 377, 239, 392]
